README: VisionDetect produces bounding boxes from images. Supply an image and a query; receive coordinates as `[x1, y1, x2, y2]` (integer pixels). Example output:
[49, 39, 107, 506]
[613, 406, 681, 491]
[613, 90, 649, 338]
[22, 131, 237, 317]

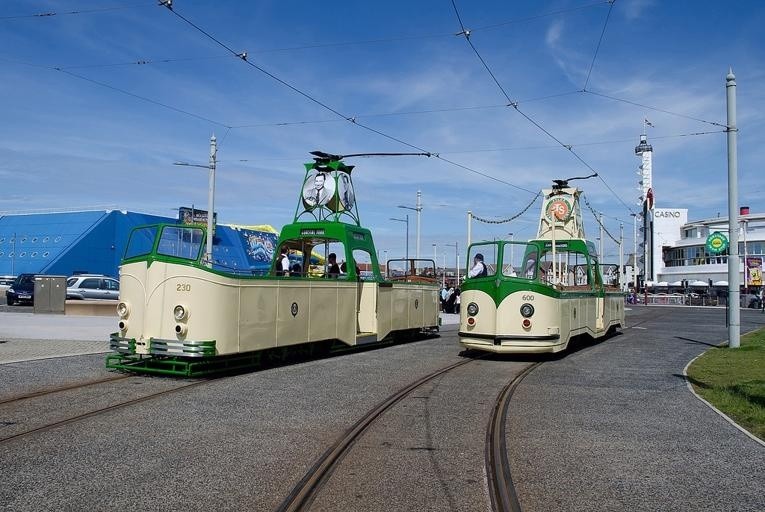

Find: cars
[0, 273, 42, 306]
[64, 273, 120, 300]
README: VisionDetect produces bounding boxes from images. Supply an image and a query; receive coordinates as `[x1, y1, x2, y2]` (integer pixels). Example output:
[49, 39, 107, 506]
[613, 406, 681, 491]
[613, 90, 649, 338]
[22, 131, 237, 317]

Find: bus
[104, 150, 441, 377]
[458, 173, 626, 354]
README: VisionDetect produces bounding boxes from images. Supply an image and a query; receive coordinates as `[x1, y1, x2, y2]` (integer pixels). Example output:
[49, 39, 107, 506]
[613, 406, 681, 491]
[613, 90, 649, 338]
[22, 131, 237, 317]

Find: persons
[276, 247, 291, 277]
[319, 253, 339, 279]
[343, 175, 354, 209]
[461, 254, 488, 279]
[440, 287, 460, 314]
[304, 172, 334, 207]
[341, 262, 361, 275]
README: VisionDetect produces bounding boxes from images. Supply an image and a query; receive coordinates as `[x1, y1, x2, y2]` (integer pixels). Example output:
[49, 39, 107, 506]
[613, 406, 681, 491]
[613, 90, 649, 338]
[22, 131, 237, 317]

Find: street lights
[388, 214, 409, 275]
[172, 205, 194, 259]
[170, 133, 218, 271]
[390, 190, 423, 278]
